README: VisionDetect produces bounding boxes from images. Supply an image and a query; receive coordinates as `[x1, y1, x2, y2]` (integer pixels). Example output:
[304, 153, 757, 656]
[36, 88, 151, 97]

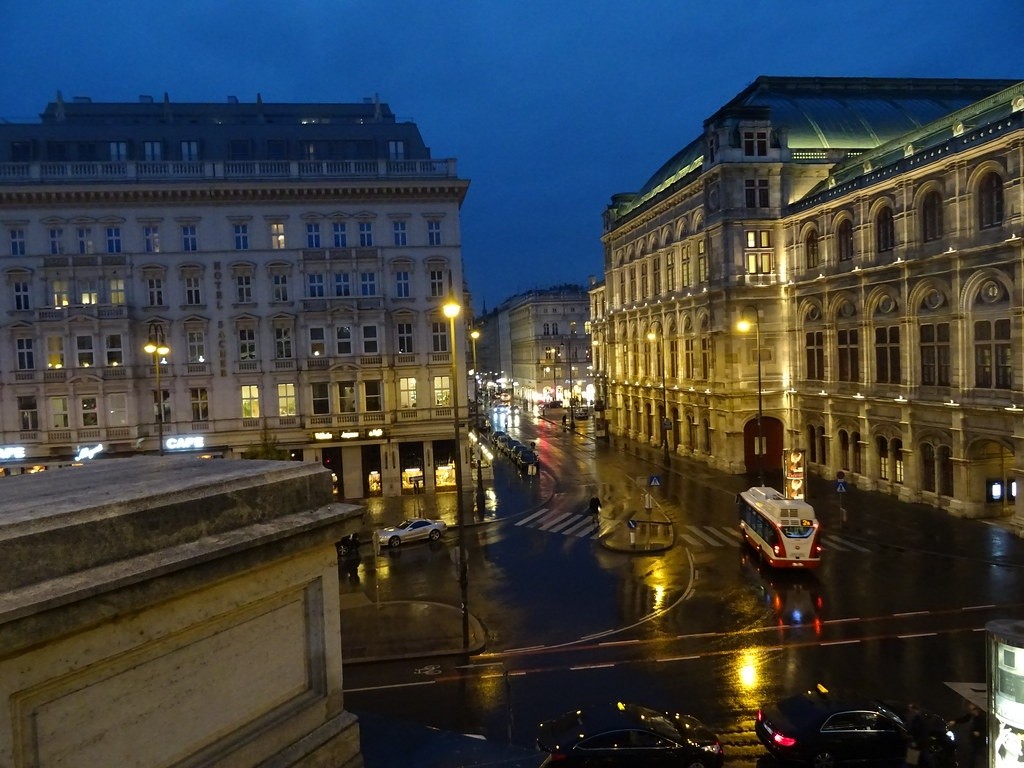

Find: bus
[731, 484, 820, 577]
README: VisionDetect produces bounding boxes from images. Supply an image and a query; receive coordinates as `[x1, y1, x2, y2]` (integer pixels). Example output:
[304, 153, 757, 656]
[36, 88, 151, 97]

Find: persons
[563, 415, 566, 425]
[589, 494, 602, 528]
[949, 705, 986, 746]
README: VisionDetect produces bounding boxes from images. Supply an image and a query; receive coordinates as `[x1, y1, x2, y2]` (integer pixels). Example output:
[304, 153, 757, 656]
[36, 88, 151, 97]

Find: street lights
[144, 321, 170, 455]
[648, 320, 669, 458]
[737, 302, 768, 484]
[470, 328, 480, 427]
[443, 288, 470, 648]
[560, 334, 575, 429]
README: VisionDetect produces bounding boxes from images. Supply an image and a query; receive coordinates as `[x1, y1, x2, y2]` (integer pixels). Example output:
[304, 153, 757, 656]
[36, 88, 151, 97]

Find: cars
[374, 515, 447, 548]
[575, 408, 588, 421]
[533, 700, 725, 768]
[754, 682, 958, 767]
[478, 389, 564, 473]
